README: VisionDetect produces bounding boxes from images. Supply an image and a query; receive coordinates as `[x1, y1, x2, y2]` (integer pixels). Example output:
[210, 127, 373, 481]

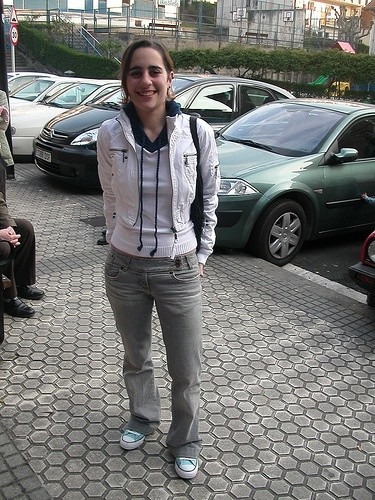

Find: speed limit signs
[10, 25, 19, 46]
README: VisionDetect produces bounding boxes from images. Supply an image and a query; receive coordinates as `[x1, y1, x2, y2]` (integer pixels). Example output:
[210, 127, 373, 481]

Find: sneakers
[120, 429, 145, 449]
[174, 456, 199, 479]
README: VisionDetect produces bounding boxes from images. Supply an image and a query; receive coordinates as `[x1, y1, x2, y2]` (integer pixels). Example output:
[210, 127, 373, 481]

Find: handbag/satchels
[189, 112, 204, 251]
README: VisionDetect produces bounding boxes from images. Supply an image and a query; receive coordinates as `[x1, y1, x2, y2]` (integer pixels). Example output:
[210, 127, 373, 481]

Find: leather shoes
[18, 288, 44, 299]
[4, 297, 35, 317]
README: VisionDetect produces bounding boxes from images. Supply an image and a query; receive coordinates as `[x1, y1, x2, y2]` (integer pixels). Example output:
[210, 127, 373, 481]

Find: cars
[5, 71, 123, 159]
[32, 73, 304, 193]
[192, 98, 375, 268]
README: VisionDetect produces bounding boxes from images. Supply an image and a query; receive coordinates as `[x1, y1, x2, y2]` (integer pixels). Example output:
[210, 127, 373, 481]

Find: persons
[0, 88, 43, 317]
[96, 38, 220, 478]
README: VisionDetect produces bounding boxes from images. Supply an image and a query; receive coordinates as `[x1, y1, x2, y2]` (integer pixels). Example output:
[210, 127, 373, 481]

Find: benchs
[149, 22, 179, 30]
[245, 32, 268, 39]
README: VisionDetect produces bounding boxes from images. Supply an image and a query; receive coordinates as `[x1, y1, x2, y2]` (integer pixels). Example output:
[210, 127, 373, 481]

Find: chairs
[340, 120, 375, 158]
